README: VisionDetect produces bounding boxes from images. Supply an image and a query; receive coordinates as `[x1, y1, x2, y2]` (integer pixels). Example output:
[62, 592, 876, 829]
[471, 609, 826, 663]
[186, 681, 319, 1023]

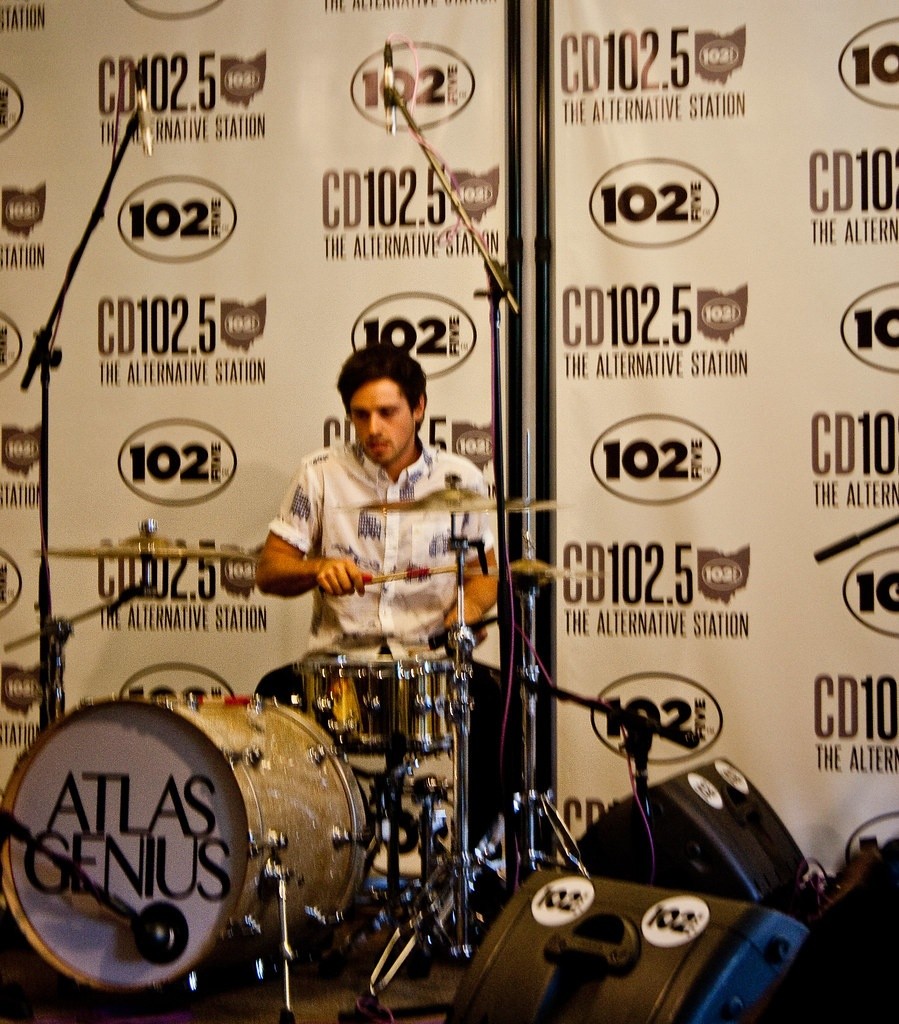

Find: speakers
[572, 756, 817, 923]
[446, 871, 811, 1024]
[752, 839, 899, 1024]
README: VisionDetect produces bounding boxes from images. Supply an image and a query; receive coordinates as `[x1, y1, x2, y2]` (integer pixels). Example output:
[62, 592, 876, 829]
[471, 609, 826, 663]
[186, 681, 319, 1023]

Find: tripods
[314, 95, 594, 1024]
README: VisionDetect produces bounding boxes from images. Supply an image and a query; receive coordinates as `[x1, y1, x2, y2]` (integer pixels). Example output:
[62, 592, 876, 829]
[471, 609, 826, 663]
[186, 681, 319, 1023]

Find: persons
[254, 345, 531, 928]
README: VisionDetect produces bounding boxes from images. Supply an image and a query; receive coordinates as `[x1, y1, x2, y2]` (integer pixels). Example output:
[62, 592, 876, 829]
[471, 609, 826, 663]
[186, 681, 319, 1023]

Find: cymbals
[356, 475, 568, 517]
[457, 558, 608, 595]
[29, 519, 262, 567]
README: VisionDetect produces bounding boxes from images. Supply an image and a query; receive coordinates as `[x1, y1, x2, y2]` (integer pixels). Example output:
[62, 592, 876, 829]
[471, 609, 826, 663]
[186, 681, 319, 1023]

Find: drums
[289, 648, 476, 757]
[0, 688, 369, 996]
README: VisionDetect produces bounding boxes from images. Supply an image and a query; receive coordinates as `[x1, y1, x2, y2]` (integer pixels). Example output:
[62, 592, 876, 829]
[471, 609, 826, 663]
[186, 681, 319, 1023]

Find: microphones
[383, 43, 396, 134]
[429, 618, 496, 649]
[149, 926, 173, 952]
[136, 70, 153, 156]
